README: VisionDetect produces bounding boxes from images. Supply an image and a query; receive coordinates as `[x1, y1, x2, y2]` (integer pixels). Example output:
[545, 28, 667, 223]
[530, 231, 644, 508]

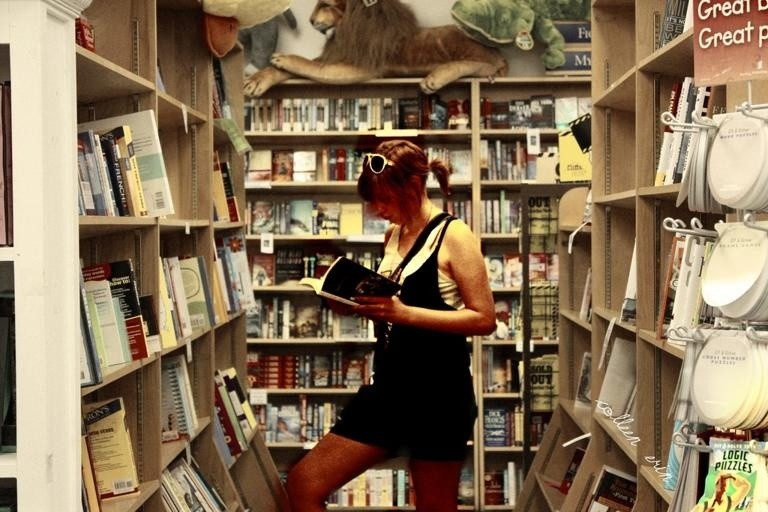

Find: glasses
[362, 153, 395, 175]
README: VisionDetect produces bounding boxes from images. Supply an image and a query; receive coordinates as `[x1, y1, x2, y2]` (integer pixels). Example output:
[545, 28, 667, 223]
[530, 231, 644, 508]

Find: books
[0, 81, 13, 245]
[555, 0, 705, 511]
[77, 108, 259, 511]
[0, 289, 16, 453]
[245, 92, 556, 509]
